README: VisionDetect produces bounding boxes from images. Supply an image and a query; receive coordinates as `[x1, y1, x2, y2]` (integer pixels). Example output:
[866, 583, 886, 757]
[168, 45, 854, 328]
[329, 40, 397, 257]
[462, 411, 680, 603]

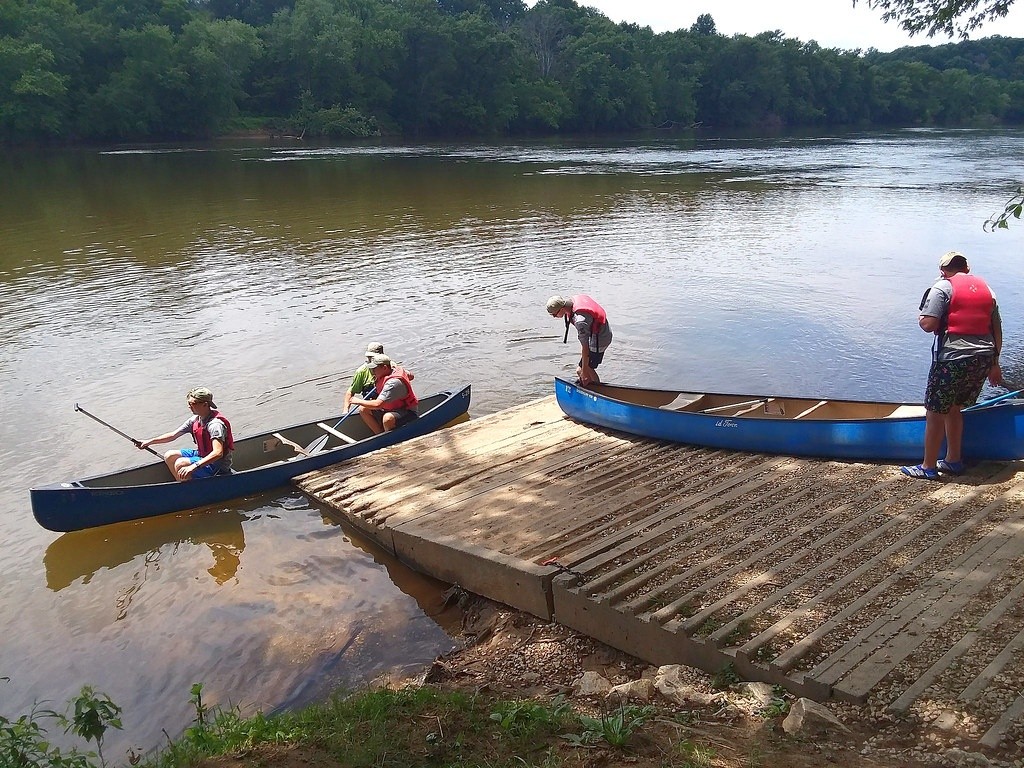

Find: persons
[546, 294, 612, 386]
[901, 251, 1002, 479]
[135, 387, 236, 482]
[343, 342, 418, 433]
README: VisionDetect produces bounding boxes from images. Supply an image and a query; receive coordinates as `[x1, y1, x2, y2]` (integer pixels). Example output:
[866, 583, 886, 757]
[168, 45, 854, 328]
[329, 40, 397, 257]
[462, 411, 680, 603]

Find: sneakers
[936, 459, 964, 474]
[901, 464, 938, 480]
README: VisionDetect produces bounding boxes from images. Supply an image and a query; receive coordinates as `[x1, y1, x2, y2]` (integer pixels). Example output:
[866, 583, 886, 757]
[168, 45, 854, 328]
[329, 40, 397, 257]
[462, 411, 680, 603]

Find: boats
[554, 376, 1024, 462]
[30, 382, 473, 533]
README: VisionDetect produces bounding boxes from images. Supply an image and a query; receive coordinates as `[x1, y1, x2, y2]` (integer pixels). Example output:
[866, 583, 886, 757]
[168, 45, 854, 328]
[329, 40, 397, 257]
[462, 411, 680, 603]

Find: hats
[547, 296, 566, 312]
[187, 387, 217, 409]
[939, 251, 967, 270]
[367, 354, 391, 369]
[365, 342, 383, 357]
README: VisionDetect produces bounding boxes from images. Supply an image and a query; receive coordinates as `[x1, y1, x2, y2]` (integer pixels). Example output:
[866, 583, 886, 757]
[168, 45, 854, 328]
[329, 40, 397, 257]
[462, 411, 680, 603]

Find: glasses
[550, 310, 560, 318]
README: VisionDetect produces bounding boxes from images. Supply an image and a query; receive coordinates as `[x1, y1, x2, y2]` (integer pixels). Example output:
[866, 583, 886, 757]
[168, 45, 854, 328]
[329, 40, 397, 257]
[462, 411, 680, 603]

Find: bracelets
[195, 462, 201, 469]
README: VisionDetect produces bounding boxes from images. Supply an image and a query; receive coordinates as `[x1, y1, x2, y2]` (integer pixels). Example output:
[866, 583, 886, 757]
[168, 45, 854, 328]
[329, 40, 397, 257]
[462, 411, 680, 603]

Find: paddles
[961, 386, 1024, 410]
[74, 402, 164, 458]
[293, 387, 378, 460]
